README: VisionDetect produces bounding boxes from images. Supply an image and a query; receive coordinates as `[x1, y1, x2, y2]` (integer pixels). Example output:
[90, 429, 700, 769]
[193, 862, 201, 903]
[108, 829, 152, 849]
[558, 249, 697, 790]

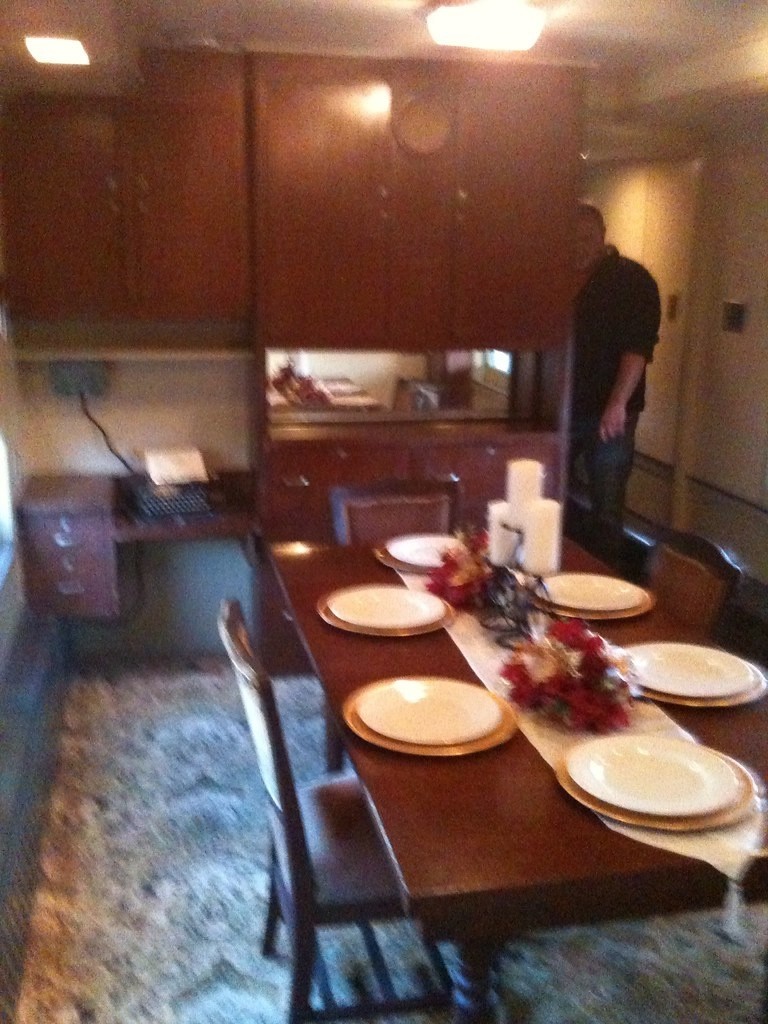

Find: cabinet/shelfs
[129, 41, 255, 350]
[242, 48, 600, 353]
[0, 86, 129, 350]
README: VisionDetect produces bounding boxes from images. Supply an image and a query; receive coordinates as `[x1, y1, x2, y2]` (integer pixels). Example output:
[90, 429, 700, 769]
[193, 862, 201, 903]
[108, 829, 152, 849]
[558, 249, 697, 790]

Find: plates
[329, 585, 444, 629]
[530, 574, 652, 620]
[373, 543, 432, 573]
[386, 534, 458, 568]
[540, 573, 646, 610]
[566, 736, 739, 818]
[358, 678, 502, 745]
[558, 748, 753, 830]
[317, 585, 455, 638]
[623, 644, 751, 696]
[342, 676, 516, 756]
[618, 648, 766, 707]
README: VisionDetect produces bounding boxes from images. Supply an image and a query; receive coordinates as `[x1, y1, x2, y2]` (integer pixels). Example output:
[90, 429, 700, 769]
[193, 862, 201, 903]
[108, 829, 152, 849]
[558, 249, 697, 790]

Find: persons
[566, 202, 662, 571]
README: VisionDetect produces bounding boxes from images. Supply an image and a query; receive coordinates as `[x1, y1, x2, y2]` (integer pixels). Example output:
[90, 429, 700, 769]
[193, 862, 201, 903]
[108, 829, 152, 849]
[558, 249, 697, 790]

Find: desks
[264, 533, 768, 1024]
[14, 471, 257, 626]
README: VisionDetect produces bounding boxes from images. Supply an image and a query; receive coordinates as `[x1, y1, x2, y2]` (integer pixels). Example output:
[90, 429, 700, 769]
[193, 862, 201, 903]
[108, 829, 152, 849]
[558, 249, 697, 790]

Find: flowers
[424, 522, 492, 617]
[499, 612, 646, 736]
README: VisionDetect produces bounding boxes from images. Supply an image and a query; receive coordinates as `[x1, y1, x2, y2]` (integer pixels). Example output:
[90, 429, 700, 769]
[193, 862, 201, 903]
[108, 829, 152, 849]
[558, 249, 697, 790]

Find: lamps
[413, 0, 563, 52]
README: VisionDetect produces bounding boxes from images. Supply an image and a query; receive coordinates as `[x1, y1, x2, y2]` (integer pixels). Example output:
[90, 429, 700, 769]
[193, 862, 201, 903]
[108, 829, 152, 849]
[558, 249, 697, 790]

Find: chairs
[329, 474, 464, 545]
[214, 596, 454, 1024]
[638, 524, 750, 641]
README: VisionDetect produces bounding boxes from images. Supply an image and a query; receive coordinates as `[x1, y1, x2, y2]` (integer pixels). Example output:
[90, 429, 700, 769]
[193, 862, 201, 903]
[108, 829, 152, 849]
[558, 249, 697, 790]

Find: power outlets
[49, 361, 116, 397]
